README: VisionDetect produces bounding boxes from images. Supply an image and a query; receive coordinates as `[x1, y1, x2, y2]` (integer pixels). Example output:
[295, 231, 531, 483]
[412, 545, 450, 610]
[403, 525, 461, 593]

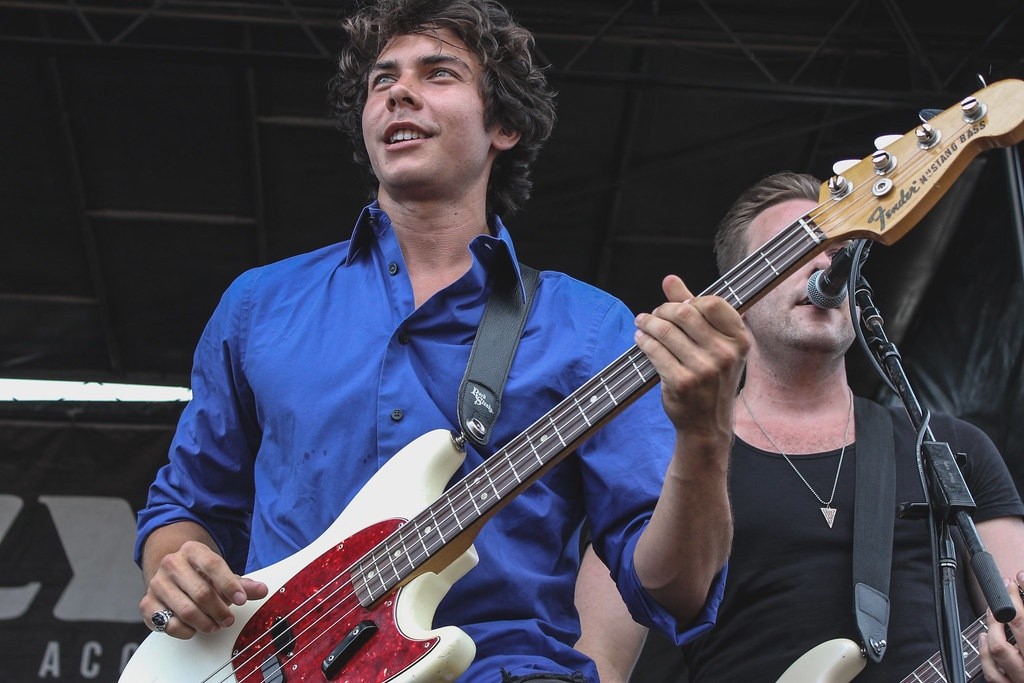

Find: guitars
[112, 67, 1023, 683]
[777, 578, 1024, 683]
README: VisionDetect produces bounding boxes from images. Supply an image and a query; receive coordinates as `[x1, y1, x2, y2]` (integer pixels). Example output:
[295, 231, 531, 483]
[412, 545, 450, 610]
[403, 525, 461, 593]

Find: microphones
[807, 237, 874, 310]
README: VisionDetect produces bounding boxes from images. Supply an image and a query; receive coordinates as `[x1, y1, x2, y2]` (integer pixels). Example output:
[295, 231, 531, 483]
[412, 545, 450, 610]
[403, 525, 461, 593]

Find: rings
[151, 609, 173, 633]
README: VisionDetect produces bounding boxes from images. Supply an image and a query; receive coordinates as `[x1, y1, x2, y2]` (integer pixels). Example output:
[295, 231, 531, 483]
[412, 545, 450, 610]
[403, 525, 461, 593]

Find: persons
[135, 0, 753, 683]
[574, 171, 1024, 683]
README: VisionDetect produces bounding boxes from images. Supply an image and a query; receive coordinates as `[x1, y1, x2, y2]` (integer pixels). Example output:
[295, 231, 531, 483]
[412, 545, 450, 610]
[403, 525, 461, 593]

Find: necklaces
[740, 385, 854, 528]
